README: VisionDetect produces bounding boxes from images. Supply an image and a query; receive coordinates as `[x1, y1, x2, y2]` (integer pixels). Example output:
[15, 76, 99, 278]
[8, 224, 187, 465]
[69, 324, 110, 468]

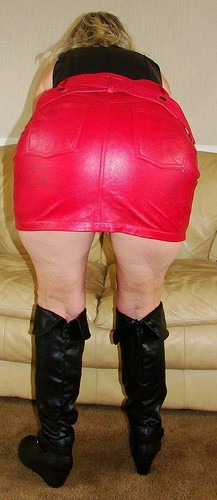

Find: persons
[13, 11, 201, 488]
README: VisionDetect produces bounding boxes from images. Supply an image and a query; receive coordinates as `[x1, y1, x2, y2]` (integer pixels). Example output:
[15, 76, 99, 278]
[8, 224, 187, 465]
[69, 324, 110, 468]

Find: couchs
[0, 144, 217, 411]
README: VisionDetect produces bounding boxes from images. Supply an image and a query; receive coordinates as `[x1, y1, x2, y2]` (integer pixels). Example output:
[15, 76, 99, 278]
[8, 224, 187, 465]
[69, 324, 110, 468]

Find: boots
[18, 304, 92, 488]
[112, 300, 169, 475]
